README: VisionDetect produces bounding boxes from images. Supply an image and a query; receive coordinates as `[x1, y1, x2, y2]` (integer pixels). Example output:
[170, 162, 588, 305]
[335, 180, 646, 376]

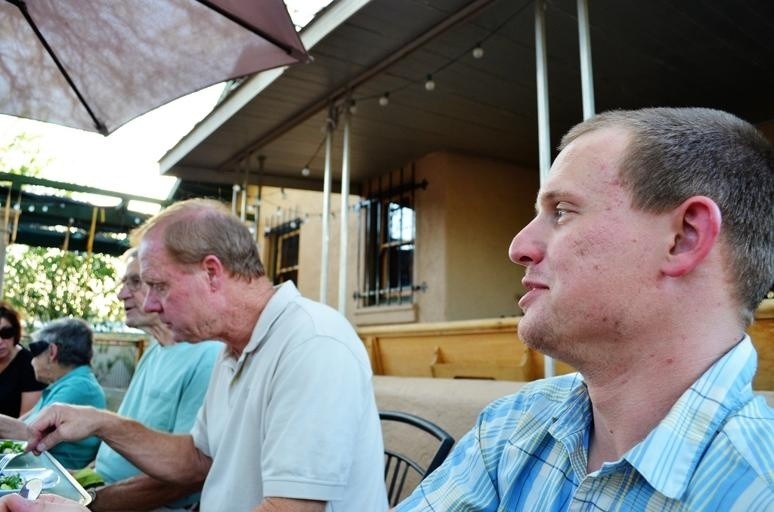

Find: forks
[0, 452, 29, 472]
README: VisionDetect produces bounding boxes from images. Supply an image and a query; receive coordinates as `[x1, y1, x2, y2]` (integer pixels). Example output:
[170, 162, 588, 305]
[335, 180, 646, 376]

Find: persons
[1, 301, 45, 421]
[0, 249, 227, 512]
[18, 195, 389, 512]
[0, 104, 774, 512]
[12, 318, 106, 471]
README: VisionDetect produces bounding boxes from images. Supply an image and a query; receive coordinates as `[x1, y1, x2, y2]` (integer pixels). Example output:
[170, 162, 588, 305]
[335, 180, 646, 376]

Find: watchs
[83, 488, 97, 512]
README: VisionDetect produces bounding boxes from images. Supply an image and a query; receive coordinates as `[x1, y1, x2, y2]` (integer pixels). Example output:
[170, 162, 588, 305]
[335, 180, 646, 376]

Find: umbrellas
[1, 0, 310, 137]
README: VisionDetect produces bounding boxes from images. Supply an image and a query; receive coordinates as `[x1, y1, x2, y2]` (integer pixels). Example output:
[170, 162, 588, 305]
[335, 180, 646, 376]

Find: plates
[0, 442, 23, 457]
[0, 468, 60, 494]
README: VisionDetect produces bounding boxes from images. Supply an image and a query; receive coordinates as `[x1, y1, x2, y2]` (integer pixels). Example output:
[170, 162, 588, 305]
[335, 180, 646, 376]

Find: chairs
[376, 409, 454, 506]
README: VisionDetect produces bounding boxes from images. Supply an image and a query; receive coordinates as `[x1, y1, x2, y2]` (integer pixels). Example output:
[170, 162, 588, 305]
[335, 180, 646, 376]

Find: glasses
[29, 341, 49, 357]
[0, 325, 15, 339]
